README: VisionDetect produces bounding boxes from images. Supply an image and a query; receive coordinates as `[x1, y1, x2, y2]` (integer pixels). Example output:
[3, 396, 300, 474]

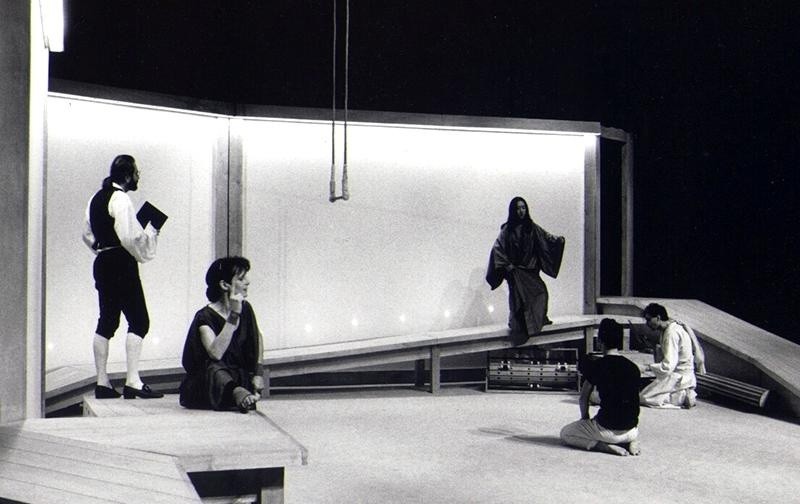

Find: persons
[485, 197, 565, 347]
[179, 256, 264, 413]
[83, 155, 163, 399]
[560, 318, 640, 457]
[639, 303, 697, 409]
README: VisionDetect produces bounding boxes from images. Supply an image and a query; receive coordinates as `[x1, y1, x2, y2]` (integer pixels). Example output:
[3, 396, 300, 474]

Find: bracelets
[227, 311, 241, 325]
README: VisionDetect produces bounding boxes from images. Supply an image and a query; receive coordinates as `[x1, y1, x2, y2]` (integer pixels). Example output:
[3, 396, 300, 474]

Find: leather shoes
[123, 383, 164, 398]
[95, 384, 121, 398]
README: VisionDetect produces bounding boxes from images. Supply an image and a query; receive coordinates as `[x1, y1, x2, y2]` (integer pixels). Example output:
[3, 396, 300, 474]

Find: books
[136, 201, 168, 229]
